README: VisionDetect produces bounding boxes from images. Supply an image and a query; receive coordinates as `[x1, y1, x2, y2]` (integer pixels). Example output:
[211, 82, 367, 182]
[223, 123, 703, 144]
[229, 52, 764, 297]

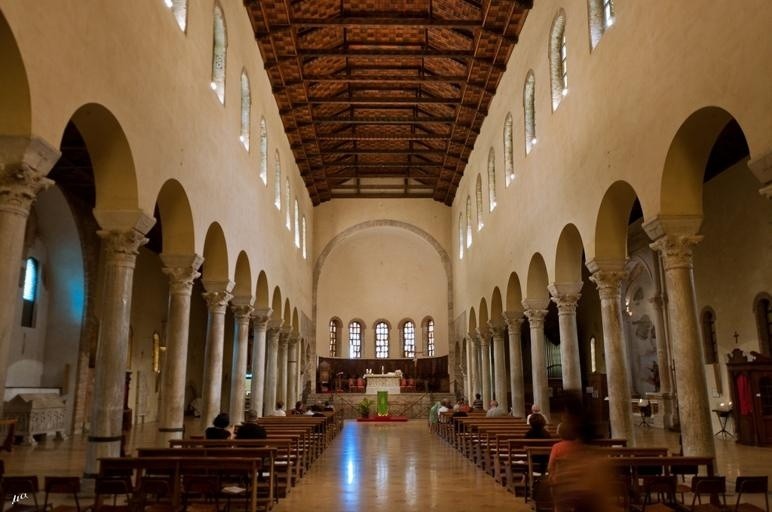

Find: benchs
[96, 406, 344, 512]
[426, 403, 714, 511]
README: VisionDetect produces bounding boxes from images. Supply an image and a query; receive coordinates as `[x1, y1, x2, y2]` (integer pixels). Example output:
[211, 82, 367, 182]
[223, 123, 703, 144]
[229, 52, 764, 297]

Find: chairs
[554, 475, 770, 512]
[399, 378, 416, 392]
[349, 377, 365, 393]
[1, 459, 220, 512]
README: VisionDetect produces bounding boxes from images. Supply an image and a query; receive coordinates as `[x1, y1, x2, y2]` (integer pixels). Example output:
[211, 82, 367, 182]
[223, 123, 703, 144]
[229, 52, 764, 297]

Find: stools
[713, 407, 735, 440]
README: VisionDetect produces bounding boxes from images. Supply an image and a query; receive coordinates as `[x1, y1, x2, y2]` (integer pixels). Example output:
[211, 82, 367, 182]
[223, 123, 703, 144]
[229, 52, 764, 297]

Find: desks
[363, 374, 402, 394]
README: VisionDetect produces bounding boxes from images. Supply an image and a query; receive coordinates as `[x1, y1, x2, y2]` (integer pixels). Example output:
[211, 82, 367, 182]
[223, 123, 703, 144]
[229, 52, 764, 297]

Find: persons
[525, 389, 631, 512]
[272, 399, 334, 433]
[234, 410, 267, 485]
[205, 412, 232, 449]
[429, 392, 506, 435]
